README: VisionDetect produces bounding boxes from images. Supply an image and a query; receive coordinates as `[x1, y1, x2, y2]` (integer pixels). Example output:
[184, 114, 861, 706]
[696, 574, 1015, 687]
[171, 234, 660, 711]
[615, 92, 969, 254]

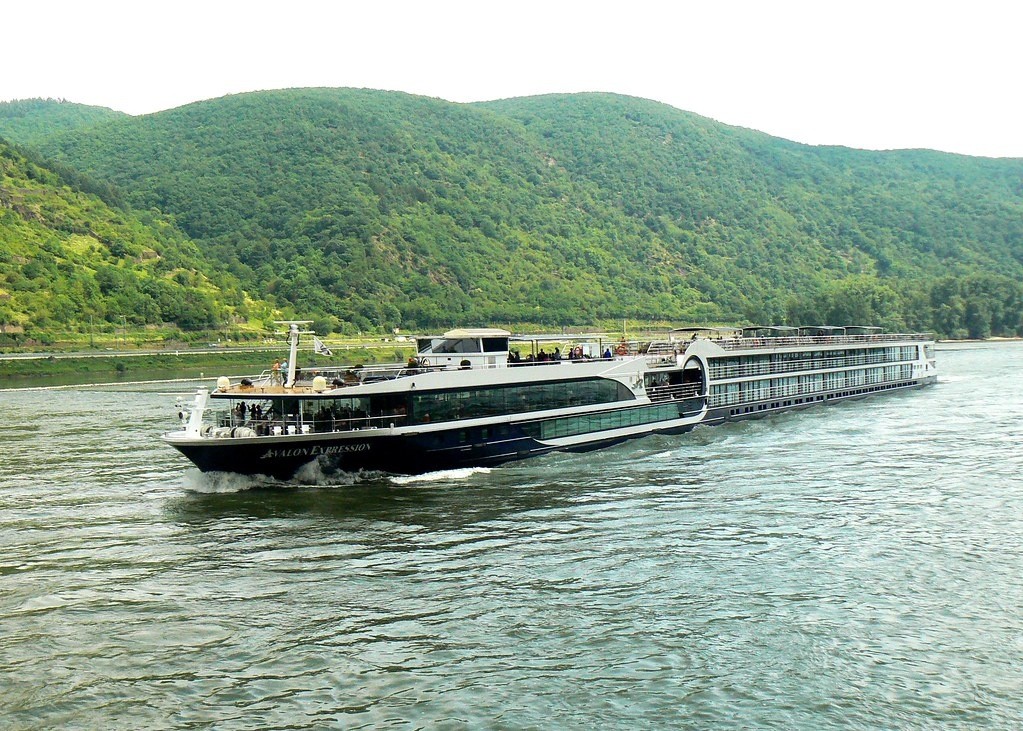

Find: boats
[161, 321, 945, 483]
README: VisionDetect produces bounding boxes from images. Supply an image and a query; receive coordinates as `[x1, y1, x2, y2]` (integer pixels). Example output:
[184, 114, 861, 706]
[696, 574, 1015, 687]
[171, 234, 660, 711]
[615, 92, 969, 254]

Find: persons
[231, 401, 263, 434]
[392, 404, 406, 425]
[692, 333, 722, 344]
[732, 331, 742, 348]
[620, 337, 626, 346]
[660, 342, 687, 364]
[295, 362, 301, 381]
[651, 378, 669, 390]
[507, 347, 561, 366]
[603, 349, 612, 361]
[816, 329, 824, 344]
[406, 357, 419, 376]
[568, 346, 592, 360]
[271, 358, 288, 385]
[752, 335, 767, 348]
[314, 403, 361, 433]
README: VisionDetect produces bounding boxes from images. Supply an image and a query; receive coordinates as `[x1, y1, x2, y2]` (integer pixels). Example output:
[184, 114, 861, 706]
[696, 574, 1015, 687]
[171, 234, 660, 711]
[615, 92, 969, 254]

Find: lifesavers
[617, 346, 626, 356]
[754, 339, 759, 348]
[574, 347, 581, 356]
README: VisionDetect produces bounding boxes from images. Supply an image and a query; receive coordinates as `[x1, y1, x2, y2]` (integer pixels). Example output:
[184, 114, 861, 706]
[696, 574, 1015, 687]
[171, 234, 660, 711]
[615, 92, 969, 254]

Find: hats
[283, 358, 287, 360]
